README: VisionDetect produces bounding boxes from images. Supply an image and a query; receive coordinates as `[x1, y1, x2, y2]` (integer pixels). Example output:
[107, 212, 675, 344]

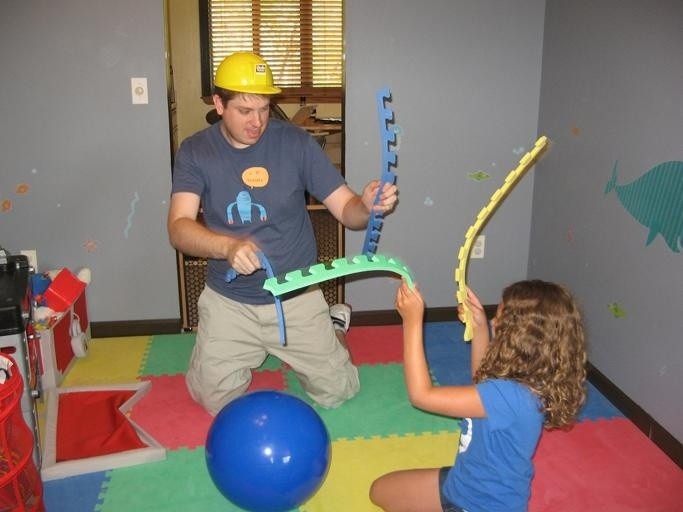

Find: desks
[299, 121, 343, 210]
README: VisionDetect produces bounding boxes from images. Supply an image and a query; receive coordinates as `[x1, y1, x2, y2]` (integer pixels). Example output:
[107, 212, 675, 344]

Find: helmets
[213, 50, 282, 95]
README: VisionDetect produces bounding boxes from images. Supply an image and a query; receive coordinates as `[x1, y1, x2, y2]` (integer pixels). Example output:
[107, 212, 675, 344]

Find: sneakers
[330, 304, 352, 335]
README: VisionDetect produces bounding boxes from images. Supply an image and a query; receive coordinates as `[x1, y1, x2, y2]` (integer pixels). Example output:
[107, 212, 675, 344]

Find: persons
[167, 52, 400, 418]
[368, 272, 589, 512]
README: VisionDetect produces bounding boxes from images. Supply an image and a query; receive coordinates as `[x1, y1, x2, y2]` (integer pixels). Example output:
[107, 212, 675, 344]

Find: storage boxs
[35, 287, 94, 391]
